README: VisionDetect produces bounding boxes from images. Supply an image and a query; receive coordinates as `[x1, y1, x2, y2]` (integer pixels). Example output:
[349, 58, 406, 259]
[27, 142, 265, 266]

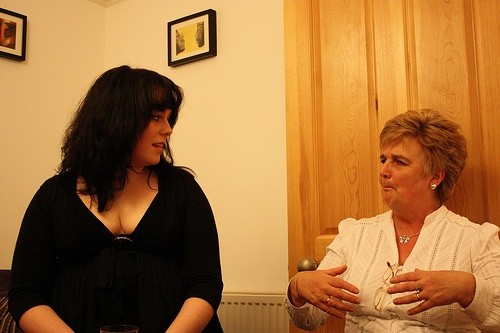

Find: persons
[286, 107, 500, 332]
[8, 64, 222, 333]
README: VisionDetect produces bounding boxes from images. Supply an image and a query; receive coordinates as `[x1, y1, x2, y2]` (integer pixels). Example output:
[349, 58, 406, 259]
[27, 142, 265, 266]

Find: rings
[417, 294, 420, 301]
[414, 281, 419, 290]
[324, 295, 330, 303]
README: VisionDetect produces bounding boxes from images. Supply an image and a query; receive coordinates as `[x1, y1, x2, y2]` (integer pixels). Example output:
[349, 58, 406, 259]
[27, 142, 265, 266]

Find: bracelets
[296, 276, 301, 298]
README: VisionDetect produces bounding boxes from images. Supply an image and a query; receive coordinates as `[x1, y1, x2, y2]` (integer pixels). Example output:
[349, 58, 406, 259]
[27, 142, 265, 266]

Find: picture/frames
[0, 7, 28, 62]
[167, 9, 217, 67]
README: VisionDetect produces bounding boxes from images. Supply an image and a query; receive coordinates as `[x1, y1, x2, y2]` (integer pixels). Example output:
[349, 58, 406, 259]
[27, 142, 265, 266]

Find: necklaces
[393, 216, 420, 243]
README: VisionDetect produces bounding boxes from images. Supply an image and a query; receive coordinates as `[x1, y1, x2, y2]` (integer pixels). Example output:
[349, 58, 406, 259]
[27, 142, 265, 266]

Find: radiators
[218, 295, 290, 333]
